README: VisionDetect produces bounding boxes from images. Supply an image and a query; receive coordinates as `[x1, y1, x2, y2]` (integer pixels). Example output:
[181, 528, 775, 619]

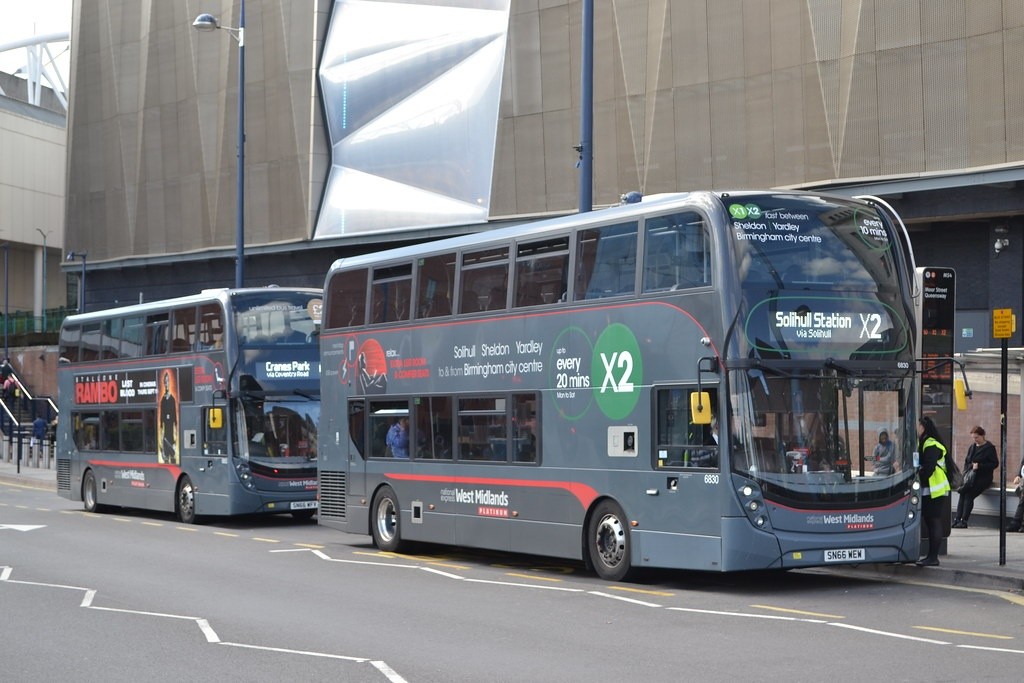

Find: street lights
[192, 0, 246, 290]
[65, 251, 86, 315]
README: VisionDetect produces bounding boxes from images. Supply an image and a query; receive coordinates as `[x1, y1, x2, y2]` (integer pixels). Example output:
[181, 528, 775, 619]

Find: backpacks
[7, 378, 17, 395]
[927, 439, 965, 490]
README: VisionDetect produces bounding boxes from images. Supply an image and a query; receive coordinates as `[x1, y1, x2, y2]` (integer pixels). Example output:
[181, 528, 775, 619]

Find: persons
[0, 359, 19, 410]
[915, 416, 952, 566]
[32, 412, 59, 458]
[1005, 456, 1024, 533]
[160, 373, 178, 464]
[952, 425, 999, 529]
[386, 416, 409, 458]
[703, 416, 744, 468]
[871, 426, 912, 478]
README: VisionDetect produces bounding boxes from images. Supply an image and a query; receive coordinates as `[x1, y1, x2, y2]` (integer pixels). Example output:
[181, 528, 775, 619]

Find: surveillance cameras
[995, 242, 1002, 251]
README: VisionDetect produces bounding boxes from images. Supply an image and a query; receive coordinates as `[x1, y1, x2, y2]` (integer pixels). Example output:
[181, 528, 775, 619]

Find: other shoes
[952, 517, 968, 529]
[916, 554, 940, 566]
[1006, 520, 1024, 533]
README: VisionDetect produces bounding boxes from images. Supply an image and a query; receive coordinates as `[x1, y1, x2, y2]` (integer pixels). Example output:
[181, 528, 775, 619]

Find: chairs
[417, 443, 535, 462]
[84, 338, 187, 360]
[348, 285, 634, 326]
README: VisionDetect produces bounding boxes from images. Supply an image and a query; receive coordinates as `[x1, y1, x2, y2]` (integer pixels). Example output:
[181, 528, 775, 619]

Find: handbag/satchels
[958, 468, 976, 493]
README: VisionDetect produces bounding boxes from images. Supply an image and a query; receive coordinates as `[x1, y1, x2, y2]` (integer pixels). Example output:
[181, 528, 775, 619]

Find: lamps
[994, 218, 1008, 257]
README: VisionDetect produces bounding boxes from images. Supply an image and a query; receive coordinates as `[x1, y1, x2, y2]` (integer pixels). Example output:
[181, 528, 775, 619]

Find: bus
[56, 283, 325, 524]
[315, 188, 973, 582]
